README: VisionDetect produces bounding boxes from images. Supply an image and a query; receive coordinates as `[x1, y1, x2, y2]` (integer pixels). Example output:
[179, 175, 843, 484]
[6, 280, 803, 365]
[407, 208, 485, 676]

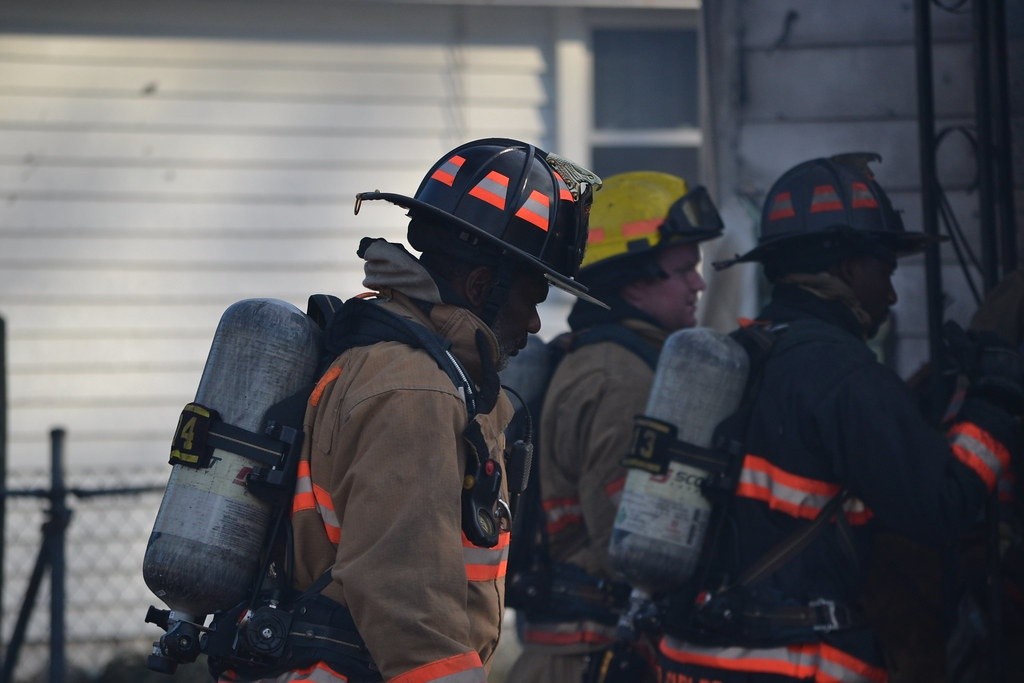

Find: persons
[509, 148, 1024, 682]
[285, 136, 606, 683]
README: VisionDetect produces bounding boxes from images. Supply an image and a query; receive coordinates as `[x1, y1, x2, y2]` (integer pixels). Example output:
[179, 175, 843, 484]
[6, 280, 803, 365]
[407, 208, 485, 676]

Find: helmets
[576, 170, 725, 274]
[354, 137, 612, 312]
[710, 151, 952, 272]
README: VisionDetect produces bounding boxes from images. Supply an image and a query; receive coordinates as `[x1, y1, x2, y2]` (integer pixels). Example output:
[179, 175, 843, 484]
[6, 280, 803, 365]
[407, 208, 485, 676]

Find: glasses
[656, 184, 724, 251]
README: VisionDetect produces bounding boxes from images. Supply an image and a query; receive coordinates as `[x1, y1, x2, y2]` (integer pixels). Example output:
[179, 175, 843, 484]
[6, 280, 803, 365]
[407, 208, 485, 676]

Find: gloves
[935, 319, 1024, 418]
